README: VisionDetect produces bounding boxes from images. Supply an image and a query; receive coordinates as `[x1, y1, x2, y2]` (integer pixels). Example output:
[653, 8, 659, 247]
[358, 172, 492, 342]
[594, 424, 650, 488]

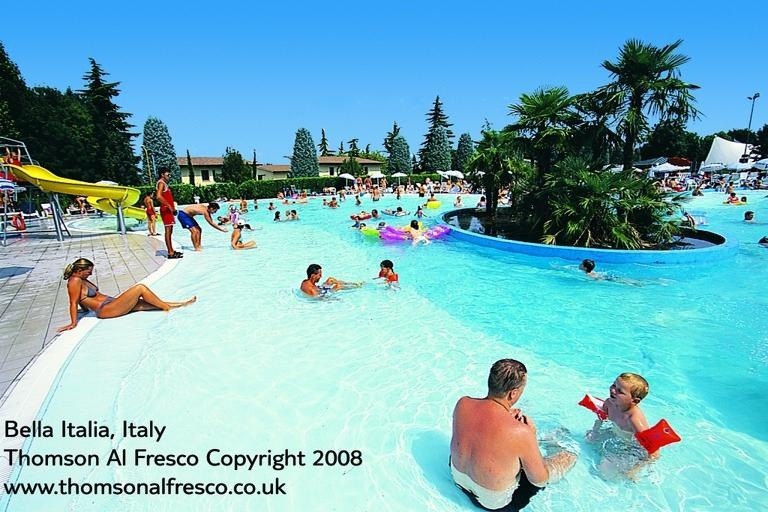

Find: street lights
[743, 93, 759, 164]
[140, 145, 153, 186]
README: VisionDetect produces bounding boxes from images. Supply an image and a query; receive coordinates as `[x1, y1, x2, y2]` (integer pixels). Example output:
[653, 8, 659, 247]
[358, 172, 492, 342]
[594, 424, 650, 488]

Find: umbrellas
[339, 169, 485, 186]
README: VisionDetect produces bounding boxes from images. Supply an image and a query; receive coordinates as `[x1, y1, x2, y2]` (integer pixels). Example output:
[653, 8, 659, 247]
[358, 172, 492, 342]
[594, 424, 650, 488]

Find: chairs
[653, 171, 767, 189]
[277, 177, 478, 196]
[39, 198, 110, 220]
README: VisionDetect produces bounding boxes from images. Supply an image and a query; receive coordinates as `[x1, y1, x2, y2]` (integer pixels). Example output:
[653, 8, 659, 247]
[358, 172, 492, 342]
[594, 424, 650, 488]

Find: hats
[237, 219, 245, 225]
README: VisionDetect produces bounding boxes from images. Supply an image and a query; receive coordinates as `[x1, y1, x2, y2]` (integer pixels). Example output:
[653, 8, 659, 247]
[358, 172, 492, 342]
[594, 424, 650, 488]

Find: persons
[584, 372, 661, 484]
[217, 174, 512, 250]
[0, 145, 22, 207]
[66, 196, 92, 216]
[371, 260, 400, 292]
[575, 259, 644, 288]
[448, 359, 577, 512]
[143, 190, 161, 236]
[300, 264, 361, 305]
[661, 172, 768, 251]
[55, 258, 198, 333]
[155, 167, 184, 259]
[178, 202, 228, 251]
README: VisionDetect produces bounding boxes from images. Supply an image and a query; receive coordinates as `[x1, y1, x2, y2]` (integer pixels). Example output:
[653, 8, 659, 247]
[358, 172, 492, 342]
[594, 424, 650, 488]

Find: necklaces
[492, 398, 509, 413]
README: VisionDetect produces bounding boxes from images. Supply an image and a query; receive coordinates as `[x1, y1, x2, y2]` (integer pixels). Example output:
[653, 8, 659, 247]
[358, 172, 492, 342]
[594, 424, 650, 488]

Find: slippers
[168, 253, 182, 258]
[174, 251, 182, 255]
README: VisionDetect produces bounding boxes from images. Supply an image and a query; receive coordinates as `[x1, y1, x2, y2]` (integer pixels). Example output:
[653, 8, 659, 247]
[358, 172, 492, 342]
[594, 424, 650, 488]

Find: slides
[1, 164, 162, 221]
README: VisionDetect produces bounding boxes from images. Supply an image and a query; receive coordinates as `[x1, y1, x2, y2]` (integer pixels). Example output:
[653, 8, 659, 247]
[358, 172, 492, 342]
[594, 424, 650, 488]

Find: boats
[421, 200, 443, 209]
[359, 220, 424, 238]
[378, 222, 449, 242]
